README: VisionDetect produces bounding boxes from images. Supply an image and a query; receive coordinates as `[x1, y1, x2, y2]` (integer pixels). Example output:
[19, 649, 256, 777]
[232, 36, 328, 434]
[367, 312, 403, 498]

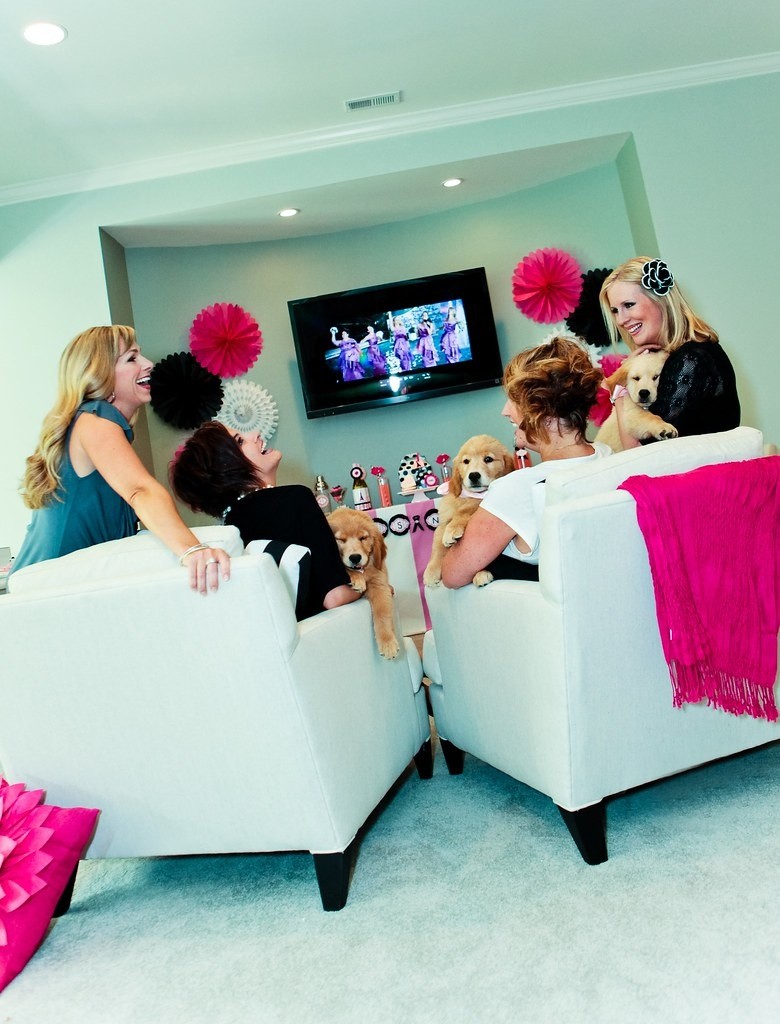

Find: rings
[207, 558, 216, 565]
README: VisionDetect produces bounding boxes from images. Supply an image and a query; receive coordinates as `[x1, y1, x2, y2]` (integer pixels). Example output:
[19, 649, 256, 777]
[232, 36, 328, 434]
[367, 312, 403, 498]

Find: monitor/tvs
[286, 266, 505, 420]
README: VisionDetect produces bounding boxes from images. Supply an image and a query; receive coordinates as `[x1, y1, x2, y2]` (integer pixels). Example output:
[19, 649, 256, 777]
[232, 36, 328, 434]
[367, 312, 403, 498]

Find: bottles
[377, 473, 392, 508]
[441, 461, 452, 483]
[352, 463, 372, 511]
[512, 436, 532, 470]
[315, 475, 333, 516]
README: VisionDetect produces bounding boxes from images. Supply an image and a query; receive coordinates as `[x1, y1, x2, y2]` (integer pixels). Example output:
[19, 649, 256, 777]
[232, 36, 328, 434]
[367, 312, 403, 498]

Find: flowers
[641, 257, 675, 296]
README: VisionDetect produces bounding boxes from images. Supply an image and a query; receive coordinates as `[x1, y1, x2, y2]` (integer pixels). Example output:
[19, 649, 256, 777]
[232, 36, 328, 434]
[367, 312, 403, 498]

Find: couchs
[0, 526, 434, 911]
[420, 426, 779, 867]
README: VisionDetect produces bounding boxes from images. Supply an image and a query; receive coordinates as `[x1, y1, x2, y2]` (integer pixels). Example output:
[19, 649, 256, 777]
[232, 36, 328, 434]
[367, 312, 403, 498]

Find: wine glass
[330, 487, 349, 512]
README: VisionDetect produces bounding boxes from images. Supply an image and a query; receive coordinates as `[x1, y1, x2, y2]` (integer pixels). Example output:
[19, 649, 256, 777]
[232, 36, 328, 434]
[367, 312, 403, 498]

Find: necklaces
[223, 482, 274, 518]
[550, 442, 582, 451]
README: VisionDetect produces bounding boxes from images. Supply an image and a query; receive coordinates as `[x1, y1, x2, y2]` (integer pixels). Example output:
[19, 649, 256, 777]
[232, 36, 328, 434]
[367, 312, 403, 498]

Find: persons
[330, 327, 366, 381]
[598, 255, 741, 451]
[387, 311, 413, 371]
[441, 336, 616, 587]
[167, 420, 394, 622]
[440, 306, 462, 364]
[416, 311, 439, 368]
[6, 325, 230, 596]
[360, 326, 391, 376]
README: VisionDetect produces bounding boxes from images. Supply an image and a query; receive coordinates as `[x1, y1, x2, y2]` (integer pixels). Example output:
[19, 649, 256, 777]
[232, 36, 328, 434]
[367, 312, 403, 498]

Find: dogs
[424, 435, 514, 590]
[326, 504, 400, 657]
[592, 352, 678, 453]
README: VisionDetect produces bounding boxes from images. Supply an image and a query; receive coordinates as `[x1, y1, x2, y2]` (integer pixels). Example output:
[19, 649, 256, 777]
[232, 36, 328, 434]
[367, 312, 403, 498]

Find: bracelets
[177, 543, 210, 567]
[608, 384, 628, 404]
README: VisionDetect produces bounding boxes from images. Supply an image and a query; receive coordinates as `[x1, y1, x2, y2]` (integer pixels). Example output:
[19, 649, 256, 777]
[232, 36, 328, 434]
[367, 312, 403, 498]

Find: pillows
[0, 779, 101, 994]
